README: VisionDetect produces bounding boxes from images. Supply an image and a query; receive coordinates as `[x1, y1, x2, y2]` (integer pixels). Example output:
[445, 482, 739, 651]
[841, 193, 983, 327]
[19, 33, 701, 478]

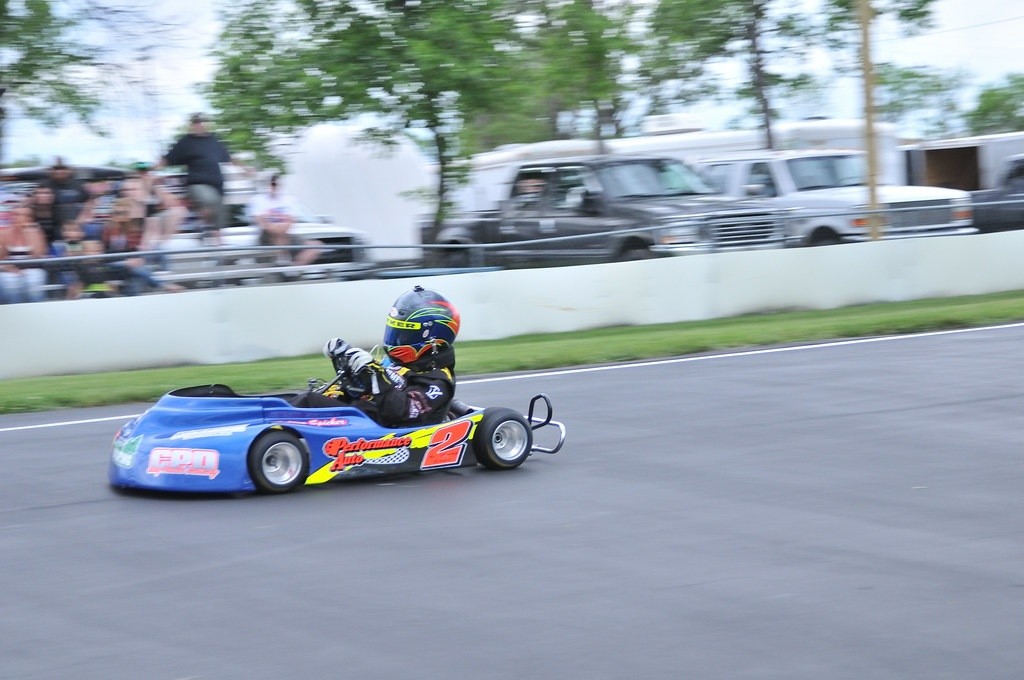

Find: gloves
[344, 347, 372, 376]
[324, 338, 350, 356]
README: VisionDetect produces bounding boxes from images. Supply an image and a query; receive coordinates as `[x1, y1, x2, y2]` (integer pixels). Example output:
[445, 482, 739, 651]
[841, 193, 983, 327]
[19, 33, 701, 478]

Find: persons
[323, 285, 460, 427]
[0, 112, 324, 304]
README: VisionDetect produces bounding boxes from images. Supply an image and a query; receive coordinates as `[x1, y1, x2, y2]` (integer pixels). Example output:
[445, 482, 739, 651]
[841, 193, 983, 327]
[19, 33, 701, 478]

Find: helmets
[383, 287, 461, 363]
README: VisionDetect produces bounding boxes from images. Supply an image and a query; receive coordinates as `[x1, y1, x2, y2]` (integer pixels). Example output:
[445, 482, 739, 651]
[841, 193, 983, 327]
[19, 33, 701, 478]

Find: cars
[973, 153, 1024, 233]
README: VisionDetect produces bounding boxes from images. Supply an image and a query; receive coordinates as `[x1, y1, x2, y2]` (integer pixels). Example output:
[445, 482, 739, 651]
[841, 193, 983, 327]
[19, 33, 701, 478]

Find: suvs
[428, 154, 788, 272]
[696, 148, 980, 249]
